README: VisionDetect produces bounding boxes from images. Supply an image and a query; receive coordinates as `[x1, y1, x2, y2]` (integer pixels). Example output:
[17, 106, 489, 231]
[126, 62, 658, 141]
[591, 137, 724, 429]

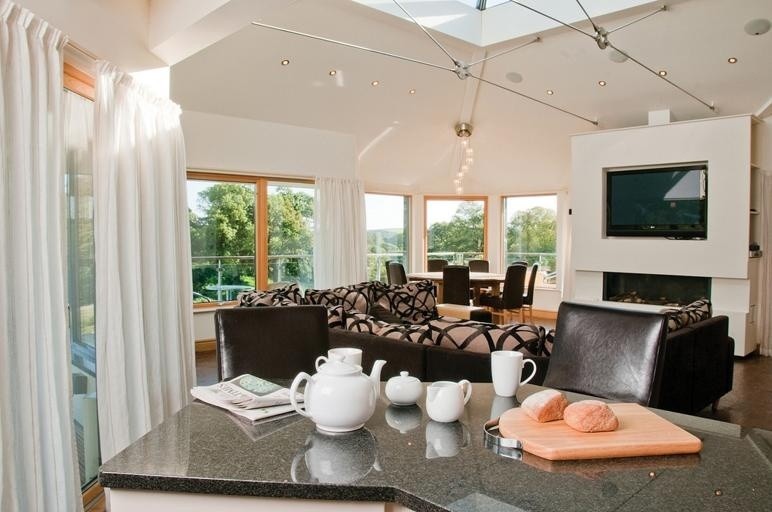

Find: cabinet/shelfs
[744, 115, 766, 354]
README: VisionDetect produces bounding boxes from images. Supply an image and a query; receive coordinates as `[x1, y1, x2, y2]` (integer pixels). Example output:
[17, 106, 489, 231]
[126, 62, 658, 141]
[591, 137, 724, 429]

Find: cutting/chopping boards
[482, 403, 703, 461]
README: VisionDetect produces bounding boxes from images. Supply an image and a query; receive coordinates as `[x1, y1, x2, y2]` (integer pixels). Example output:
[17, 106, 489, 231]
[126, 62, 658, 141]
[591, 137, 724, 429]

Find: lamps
[454, 122, 473, 197]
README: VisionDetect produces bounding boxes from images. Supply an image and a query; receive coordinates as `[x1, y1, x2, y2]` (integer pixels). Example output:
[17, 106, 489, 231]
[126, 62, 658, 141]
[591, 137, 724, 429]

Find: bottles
[426, 379, 473, 423]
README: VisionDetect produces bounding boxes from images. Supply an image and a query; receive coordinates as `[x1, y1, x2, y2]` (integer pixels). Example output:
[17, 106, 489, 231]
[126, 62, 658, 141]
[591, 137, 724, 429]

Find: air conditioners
[72, 392, 101, 485]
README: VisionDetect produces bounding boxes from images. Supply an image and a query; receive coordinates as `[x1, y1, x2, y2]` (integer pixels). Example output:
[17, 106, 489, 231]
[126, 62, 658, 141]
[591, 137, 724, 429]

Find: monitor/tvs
[605, 163, 707, 238]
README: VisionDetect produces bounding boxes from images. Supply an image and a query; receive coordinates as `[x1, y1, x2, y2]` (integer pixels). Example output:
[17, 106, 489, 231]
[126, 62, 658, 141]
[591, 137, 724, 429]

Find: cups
[315, 348, 363, 372]
[490, 350, 537, 397]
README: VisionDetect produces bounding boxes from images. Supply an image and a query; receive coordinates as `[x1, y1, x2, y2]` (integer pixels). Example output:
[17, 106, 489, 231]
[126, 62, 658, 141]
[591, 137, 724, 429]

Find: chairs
[442, 265, 471, 306]
[427, 260, 448, 272]
[479, 264, 527, 324]
[542, 301, 668, 408]
[512, 262, 527, 265]
[469, 260, 489, 272]
[389, 262, 408, 284]
[216, 306, 330, 380]
[523, 265, 538, 324]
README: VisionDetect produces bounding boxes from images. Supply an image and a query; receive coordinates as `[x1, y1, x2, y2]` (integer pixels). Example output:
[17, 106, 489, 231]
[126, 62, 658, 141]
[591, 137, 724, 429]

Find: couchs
[237, 280, 734, 416]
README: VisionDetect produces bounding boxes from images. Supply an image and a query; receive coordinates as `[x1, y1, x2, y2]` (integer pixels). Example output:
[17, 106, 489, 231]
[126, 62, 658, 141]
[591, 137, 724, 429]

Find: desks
[406, 272, 505, 296]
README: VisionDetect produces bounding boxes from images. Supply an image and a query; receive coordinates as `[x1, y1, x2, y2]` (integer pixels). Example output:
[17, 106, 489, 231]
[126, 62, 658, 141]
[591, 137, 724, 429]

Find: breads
[563, 400, 618, 432]
[521, 388, 567, 423]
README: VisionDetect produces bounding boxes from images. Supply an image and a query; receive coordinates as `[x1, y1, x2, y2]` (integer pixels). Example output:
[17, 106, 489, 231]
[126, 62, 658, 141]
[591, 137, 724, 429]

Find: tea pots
[290, 356, 387, 433]
